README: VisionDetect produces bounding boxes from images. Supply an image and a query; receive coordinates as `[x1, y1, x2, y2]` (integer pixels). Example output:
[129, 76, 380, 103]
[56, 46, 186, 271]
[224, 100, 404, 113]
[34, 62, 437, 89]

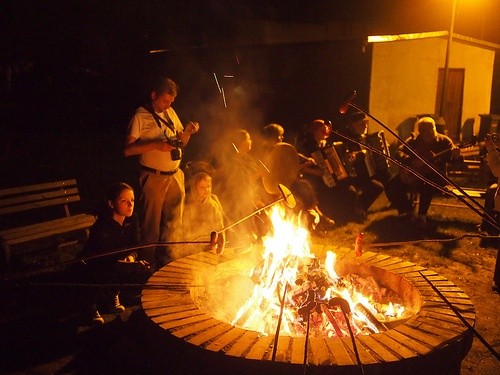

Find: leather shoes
[140, 165, 177, 175]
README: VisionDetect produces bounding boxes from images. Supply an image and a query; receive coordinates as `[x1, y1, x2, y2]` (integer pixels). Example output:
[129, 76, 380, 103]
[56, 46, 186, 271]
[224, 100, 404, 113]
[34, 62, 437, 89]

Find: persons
[124, 79, 200, 265]
[485, 118, 500, 294]
[384, 117, 464, 228]
[182, 111, 384, 260]
[61, 183, 150, 326]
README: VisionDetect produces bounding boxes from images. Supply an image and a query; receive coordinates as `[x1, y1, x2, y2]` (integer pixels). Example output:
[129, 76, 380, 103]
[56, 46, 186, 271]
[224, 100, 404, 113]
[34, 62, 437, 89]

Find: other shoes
[476, 221, 500, 237]
[354, 205, 367, 217]
[408, 207, 427, 226]
[313, 215, 335, 238]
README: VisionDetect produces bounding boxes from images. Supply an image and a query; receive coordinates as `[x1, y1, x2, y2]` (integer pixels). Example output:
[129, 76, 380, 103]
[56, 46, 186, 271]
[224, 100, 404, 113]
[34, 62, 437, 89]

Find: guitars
[394, 140, 476, 185]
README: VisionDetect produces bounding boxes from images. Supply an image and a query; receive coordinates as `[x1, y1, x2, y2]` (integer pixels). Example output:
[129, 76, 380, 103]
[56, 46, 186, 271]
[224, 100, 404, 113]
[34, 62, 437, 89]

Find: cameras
[166, 141, 182, 160]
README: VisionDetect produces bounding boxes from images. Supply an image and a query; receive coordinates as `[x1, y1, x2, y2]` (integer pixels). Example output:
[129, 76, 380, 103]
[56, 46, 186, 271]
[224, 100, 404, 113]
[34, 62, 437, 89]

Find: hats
[351, 111, 370, 121]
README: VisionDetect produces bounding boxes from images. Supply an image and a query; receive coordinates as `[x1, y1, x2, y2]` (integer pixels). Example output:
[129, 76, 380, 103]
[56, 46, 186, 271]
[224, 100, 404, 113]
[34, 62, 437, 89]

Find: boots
[75, 297, 104, 326]
[98, 289, 125, 312]
